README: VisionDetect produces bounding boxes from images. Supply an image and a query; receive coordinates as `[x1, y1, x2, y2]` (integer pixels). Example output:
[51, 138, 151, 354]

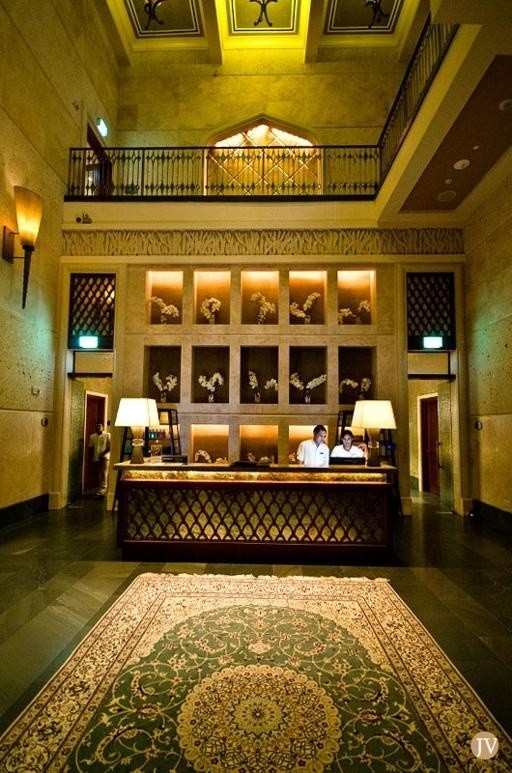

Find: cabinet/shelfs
[113, 410, 180, 462]
[335, 410, 397, 466]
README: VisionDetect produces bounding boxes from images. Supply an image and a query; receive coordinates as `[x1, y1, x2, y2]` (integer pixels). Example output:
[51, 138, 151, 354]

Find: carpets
[2, 572, 509, 773]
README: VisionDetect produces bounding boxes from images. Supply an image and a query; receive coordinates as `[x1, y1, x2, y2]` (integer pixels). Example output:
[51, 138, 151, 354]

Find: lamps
[351, 399, 397, 467]
[114, 396, 159, 464]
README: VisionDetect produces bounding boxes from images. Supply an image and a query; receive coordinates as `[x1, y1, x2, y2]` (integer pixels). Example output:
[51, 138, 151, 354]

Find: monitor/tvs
[329, 456, 366, 465]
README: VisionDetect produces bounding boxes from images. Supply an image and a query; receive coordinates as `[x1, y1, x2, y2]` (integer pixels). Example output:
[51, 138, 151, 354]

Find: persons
[329, 430, 368, 464]
[296, 425, 331, 469]
[88, 423, 111, 495]
